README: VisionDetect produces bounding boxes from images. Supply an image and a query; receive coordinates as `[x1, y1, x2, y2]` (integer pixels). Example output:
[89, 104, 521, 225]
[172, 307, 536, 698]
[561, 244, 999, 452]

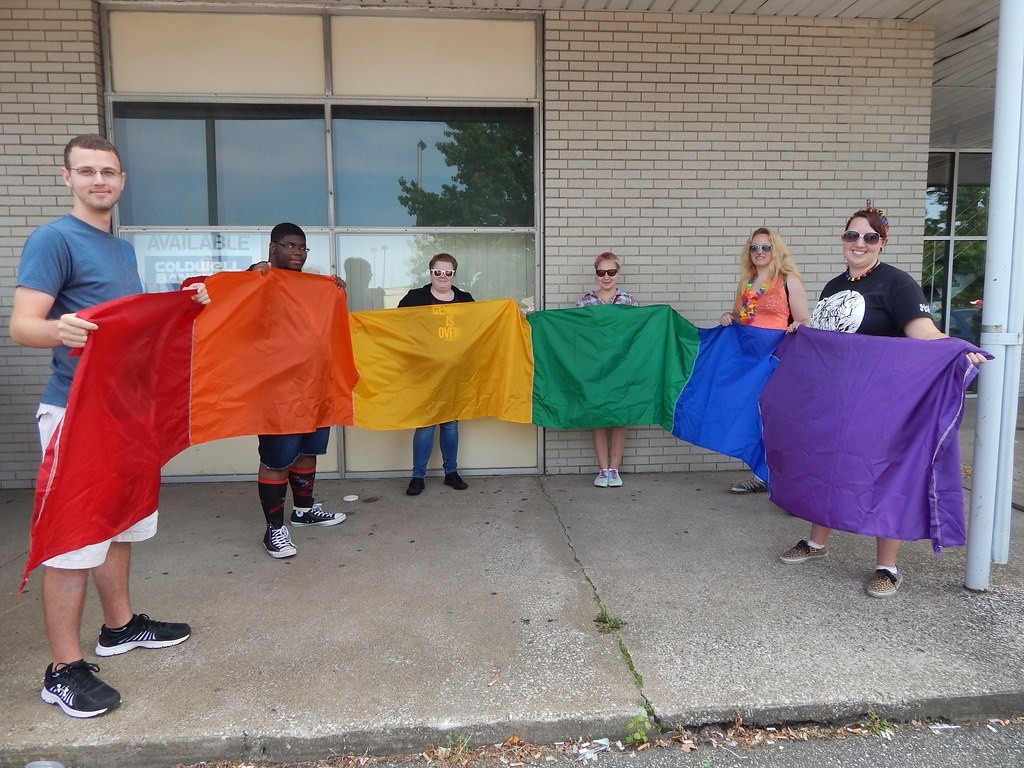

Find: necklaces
[739, 275, 771, 323]
[846, 259, 878, 281]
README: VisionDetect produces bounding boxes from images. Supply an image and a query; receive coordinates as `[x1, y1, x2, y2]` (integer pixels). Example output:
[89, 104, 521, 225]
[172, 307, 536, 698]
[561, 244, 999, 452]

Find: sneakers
[407, 478, 425, 495]
[445, 471, 469, 490]
[263, 523, 298, 558]
[779, 539, 828, 565]
[290, 503, 346, 527]
[729, 477, 768, 495]
[608, 467, 622, 486]
[865, 564, 903, 599]
[41, 658, 120, 718]
[95, 613, 191, 656]
[594, 468, 609, 487]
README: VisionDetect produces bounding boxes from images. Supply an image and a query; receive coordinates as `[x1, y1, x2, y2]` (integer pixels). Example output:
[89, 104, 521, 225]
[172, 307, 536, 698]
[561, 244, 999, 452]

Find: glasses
[596, 269, 618, 277]
[272, 240, 310, 253]
[431, 268, 456, 278]
[841, 230, 884, 245]
[67, 167, 122, 179]
[749, 244, 772, 253]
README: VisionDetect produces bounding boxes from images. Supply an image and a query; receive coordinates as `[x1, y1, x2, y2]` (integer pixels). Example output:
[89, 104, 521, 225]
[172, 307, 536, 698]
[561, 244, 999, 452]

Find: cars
[927, 309, 982, 348]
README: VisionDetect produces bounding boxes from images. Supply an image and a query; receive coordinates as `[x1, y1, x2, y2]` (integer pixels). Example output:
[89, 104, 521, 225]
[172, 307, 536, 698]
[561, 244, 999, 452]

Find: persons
[9, 135, 191, 717]
[397, 254, 475, 495]
[247, 222, 346, 558]
[576, 253, 638, 487]
[720, 227, 810, 494]
[779, 211, 987, 598]
